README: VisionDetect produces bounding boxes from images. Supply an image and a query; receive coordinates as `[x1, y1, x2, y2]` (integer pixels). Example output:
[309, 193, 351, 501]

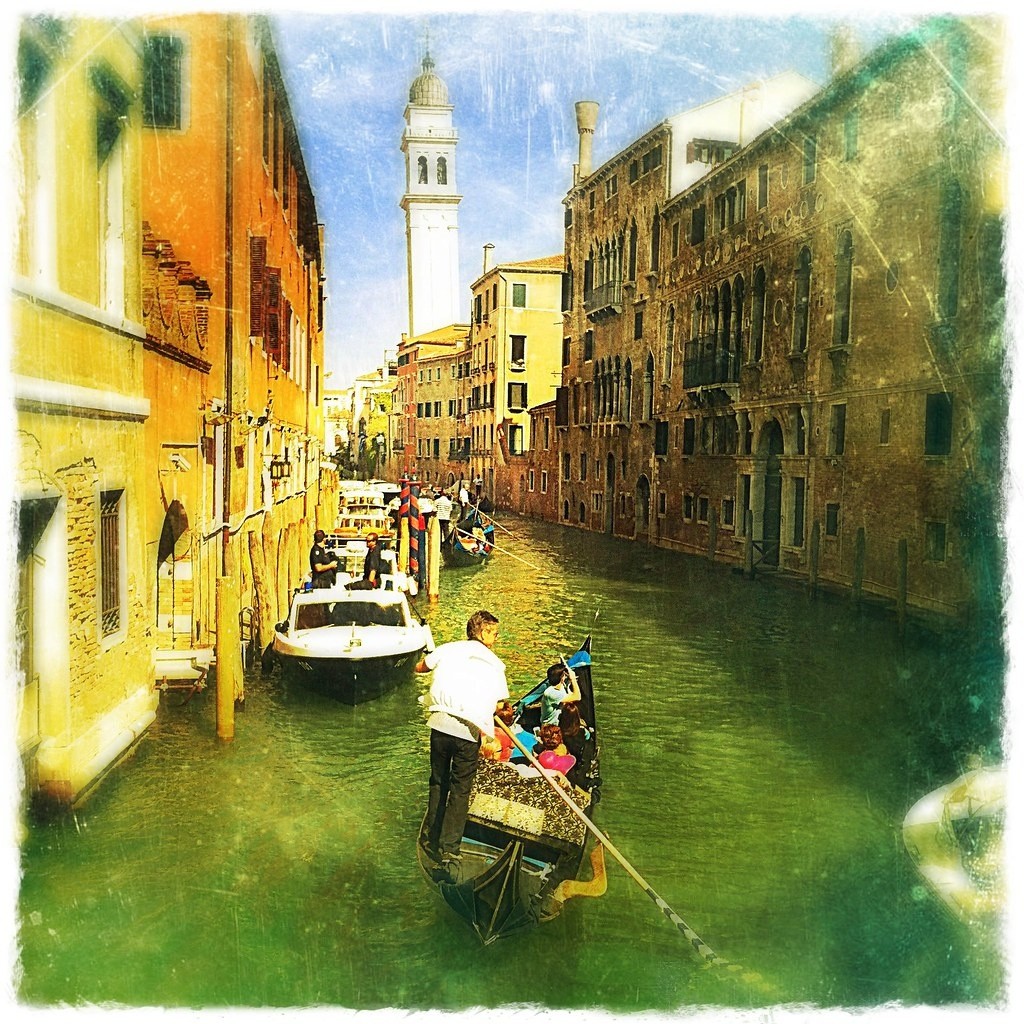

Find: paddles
[455, 526, 539, 569]
[406, 593, 427, 626]
[466, 501, 514, 536]
[493, 711, 717, 961]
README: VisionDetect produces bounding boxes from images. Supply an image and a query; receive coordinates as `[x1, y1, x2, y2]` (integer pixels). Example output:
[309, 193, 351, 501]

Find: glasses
[497, 747, 503, 753]
[366, 538, 375, 542]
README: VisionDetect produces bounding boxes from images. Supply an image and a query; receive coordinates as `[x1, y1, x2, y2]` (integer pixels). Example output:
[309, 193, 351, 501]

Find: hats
[529, 751, 577, 777]
[481, 726, 516, 749]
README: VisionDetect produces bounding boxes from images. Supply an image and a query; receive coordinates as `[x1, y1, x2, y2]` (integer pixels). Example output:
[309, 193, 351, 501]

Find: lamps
[280, 455, 290, 477]
[261, 454, 282, 479]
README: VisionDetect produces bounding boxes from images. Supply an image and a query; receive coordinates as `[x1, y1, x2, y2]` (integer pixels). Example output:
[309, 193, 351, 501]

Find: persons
[413, 612, 513, 863]
[419, 474, 484, 543]
[539, 725, 567, 756]
[310, 530, 338, 589]
[539, 664, 582, 724]
[558, 704, 587, 771]
[477, 698, 515, 762]
[363, 532, 382, 589]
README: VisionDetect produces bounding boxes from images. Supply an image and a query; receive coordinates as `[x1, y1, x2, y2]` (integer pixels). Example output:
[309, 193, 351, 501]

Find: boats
[440, 506, 494, 567]
[900, 764, 1000, 960]
[417, 626, 610, 946]
[271, 474, 424, 706]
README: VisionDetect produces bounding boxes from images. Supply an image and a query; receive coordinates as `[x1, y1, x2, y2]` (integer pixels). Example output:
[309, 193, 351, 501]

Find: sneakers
[429, 841, 438, 850]
[442, 851, 464, 863]
